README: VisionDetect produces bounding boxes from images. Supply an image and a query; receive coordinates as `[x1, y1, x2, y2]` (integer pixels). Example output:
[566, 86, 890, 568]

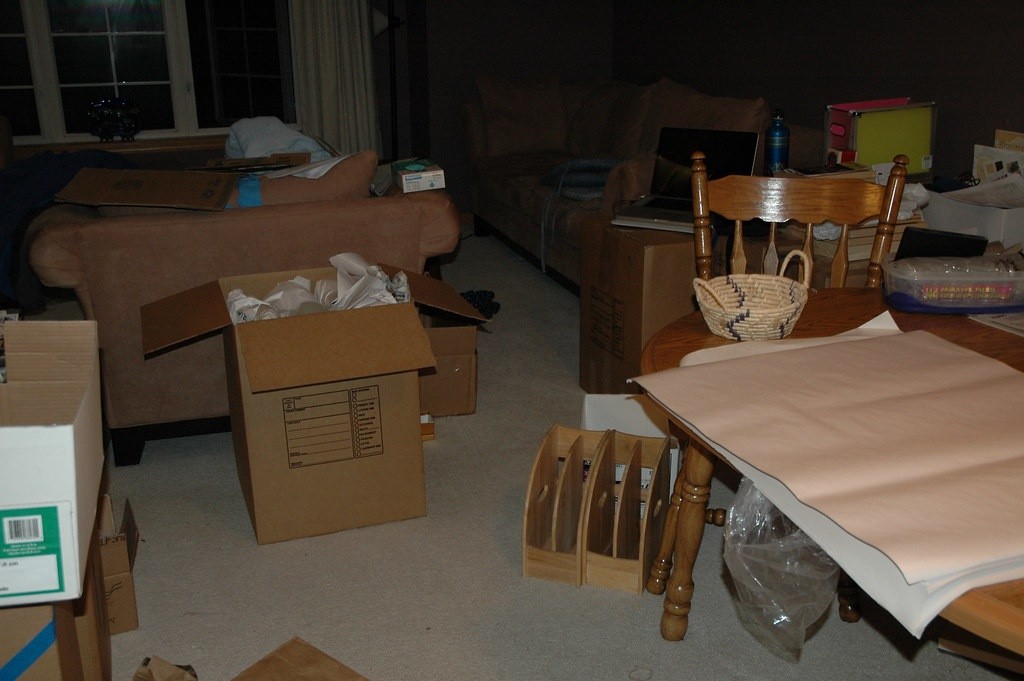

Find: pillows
[225, 151, 382, 208]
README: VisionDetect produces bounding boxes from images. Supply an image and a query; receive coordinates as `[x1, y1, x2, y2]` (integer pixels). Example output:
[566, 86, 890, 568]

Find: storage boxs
[0, 158, 497, 681]
[574, 211, 707, 396]
[823, 97, 936, 173]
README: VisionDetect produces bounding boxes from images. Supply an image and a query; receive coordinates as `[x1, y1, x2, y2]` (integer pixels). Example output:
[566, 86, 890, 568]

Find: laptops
[615, 126, 759, 228]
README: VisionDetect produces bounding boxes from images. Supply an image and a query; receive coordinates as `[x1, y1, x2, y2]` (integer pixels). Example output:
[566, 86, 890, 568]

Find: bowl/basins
[881, 251, 1024, 308]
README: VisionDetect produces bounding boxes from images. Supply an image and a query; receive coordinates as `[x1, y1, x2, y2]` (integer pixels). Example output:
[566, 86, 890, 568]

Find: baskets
[693, 250, 809, 340]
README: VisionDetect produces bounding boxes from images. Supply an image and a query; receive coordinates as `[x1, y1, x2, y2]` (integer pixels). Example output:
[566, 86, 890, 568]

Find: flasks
[764, 110, 790, 178]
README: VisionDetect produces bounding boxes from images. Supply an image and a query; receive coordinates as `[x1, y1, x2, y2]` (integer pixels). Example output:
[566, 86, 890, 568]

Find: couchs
[471, 72, 828, 283]
[27, 148, 459, 470]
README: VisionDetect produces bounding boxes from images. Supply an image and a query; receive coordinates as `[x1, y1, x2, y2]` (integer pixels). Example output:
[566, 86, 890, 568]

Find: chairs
[690, 151, 909, 289]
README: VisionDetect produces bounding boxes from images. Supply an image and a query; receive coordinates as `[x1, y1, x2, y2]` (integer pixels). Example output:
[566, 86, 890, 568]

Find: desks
[640, 289, 1024, 659]
